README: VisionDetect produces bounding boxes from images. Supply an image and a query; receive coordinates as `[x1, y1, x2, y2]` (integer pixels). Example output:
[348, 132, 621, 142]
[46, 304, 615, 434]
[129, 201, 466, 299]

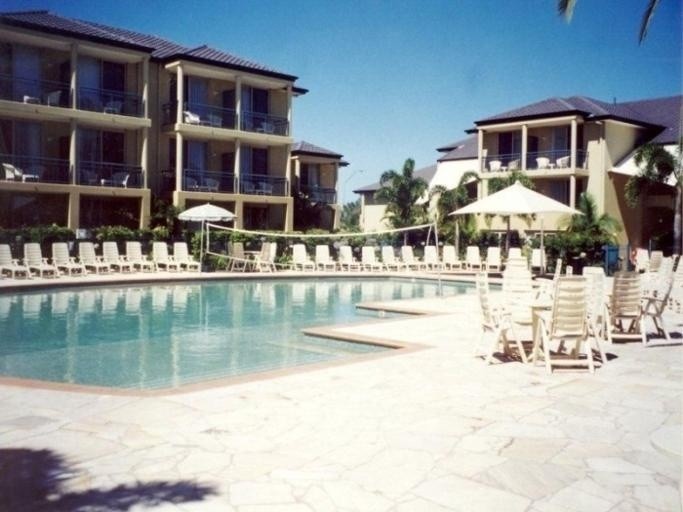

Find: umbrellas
[448, 179, 588, 246]
[176, 201, 240, 263]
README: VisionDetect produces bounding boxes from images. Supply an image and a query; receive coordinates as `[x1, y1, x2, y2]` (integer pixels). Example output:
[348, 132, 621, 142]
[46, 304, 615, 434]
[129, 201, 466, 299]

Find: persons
[649, 214, 669, 250]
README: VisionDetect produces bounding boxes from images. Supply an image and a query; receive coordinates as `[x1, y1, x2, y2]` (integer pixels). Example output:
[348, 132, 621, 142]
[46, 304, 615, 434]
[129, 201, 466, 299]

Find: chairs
[22, 88, 62, 108]
[182, 176, 220, 192]
[487, 153, 569, 173]
[1, 234, 201, 280]
[464, 243, 683, 375]
[80, 168, 129, 188]
[101, 100, 121, 114]
[184, 109, 223, 127]
[3, 161, 46, 182]
[243, 118, 276, 134]
[239, 180, 274, 195]
[226, 239, 462, 273]
